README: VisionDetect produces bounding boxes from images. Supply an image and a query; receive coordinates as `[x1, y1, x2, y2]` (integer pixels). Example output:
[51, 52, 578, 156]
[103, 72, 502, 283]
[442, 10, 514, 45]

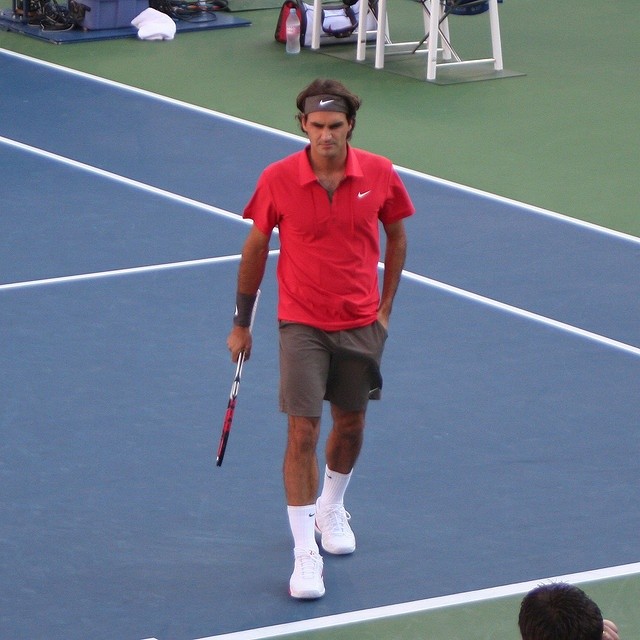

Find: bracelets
[233, 292, 256, 327]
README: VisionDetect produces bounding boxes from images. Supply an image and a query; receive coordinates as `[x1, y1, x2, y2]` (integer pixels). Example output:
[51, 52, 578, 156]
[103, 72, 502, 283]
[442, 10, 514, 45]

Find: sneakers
[315, 498, 357, 556]
[288, 546, 327, 600]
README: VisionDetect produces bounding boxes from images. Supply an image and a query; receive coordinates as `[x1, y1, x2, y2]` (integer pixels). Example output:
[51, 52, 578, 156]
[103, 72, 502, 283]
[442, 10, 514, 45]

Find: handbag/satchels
[274, 1, 379, 46]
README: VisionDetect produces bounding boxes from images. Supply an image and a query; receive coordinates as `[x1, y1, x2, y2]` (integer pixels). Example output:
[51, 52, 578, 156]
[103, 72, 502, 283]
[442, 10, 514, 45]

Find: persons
[227, 76, 416, 600]
[518, 580, 619, 640]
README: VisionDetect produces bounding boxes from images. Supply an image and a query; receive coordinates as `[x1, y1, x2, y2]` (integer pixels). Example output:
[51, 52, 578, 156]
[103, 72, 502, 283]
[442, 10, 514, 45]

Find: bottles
[285, 8, 301, 55]
